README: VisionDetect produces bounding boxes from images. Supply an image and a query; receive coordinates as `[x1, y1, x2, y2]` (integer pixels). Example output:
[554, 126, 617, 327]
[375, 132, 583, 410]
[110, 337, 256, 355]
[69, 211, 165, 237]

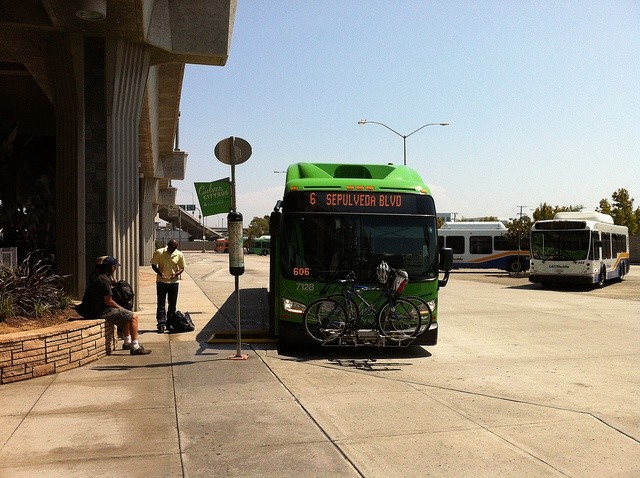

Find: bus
[215, 237, 229, 253]
[252, 235, 270, 256]
[438, 221, 525, 272]
[528, 208, 630, 287]
[269, 161, 453, 347]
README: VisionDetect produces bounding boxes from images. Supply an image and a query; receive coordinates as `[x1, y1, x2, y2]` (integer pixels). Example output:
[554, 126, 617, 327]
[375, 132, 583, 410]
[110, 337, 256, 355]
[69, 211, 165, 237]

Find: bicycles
[316, 268, 432, 340]
[302, 267, 421, 343]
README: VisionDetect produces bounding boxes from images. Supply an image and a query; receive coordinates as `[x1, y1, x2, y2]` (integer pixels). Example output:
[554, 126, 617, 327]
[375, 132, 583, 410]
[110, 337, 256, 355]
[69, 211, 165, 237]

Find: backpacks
[112, 280, 135, 310]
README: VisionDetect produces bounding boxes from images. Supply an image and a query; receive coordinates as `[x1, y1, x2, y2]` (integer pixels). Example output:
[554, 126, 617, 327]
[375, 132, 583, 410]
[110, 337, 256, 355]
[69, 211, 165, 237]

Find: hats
[95, 256, 108, 265]
[103, 256, 122, 266]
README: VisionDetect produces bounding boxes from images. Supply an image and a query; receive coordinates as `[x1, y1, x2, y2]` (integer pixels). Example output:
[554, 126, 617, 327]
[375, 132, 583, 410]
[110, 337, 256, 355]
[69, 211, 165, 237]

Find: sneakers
[130, 345, 152, 355]
[122, 343, 131, 350]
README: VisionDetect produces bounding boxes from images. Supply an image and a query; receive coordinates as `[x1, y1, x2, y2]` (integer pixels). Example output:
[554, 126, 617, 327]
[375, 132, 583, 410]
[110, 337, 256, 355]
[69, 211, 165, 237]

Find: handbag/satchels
[389, 274, 407, 295]
[171, 311, 195, 332]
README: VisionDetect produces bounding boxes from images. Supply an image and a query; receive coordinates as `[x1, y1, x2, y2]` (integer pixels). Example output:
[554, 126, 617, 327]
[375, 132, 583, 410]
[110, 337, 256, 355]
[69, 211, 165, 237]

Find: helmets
[376, 260, 390, 284]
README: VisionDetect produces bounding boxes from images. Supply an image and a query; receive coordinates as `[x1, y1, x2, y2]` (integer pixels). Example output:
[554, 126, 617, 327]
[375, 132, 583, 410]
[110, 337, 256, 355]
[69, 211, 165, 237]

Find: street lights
[358, 118, 449, 165]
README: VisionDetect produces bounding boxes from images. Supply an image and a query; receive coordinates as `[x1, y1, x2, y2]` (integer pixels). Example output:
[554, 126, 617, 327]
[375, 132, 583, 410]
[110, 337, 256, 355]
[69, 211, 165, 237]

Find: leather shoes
[158, 325, 165, 333]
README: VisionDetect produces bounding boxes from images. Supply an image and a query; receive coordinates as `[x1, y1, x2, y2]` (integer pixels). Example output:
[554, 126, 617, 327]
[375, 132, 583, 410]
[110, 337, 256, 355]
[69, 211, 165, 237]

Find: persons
[151, 239, 187, 332]
[82, 255, 130, 340]
[82, 257, 152, 355]
